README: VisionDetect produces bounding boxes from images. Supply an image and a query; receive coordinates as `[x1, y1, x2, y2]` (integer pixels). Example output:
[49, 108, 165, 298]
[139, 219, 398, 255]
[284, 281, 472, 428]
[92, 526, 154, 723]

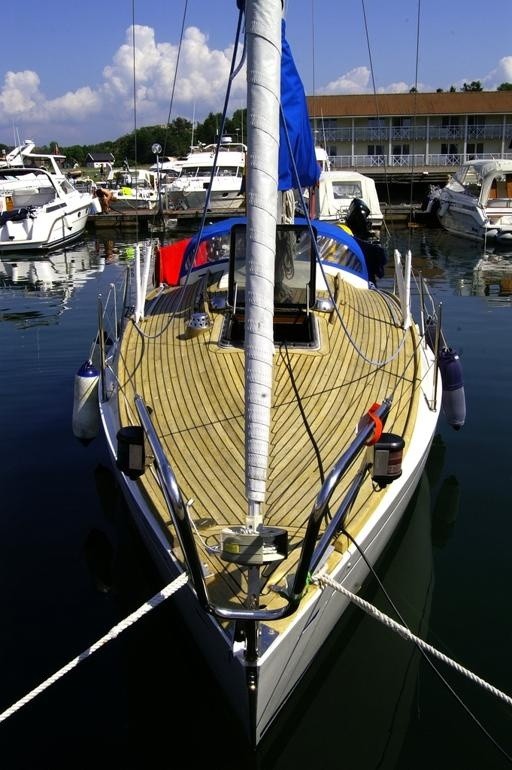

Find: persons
[72, 160, 79, 169]
[100, 156, 130, 182]
[92, 187, 113, 215]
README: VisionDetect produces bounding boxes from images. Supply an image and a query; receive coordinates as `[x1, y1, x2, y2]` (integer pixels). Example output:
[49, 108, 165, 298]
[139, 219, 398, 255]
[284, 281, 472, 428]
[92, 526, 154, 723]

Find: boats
[0, 236, 110, 322]
[0, 126, 105, 259]
[425, 152, 512, 246]
[428, 230, 512, 300]
[62, 0, 463, 757]
